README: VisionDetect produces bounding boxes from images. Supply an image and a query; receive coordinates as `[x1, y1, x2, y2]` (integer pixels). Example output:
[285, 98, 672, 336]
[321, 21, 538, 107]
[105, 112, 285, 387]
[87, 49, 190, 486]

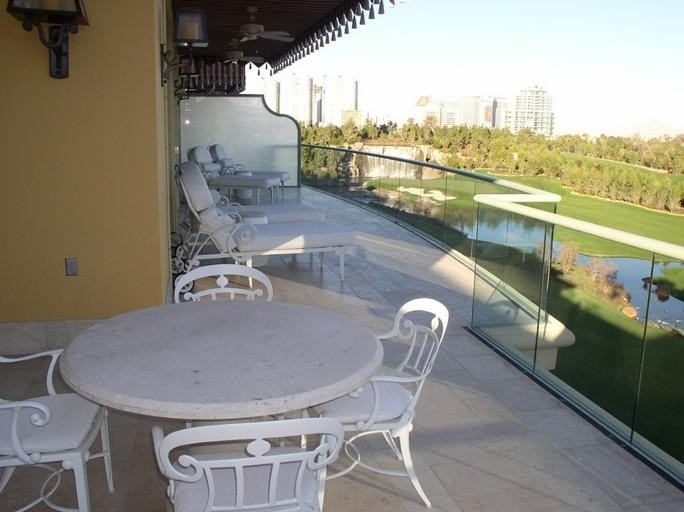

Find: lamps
[231, 58, 238, 64]
[160, 6, 207, 87]
[248, 34, 257, 40]
[174, 56, 201, 105]
[7, 0, 91, 79]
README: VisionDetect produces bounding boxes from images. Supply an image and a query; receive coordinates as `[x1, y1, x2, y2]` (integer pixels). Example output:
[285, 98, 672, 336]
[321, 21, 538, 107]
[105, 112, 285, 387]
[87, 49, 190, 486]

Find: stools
[234, 170, 254, 199]
[236, 211, 269, 268]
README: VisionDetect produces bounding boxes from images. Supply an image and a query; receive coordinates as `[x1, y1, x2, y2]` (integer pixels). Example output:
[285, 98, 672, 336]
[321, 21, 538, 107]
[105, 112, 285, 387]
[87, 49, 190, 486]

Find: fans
[222, 0, 294, 43]
[214, 32, 265, 64]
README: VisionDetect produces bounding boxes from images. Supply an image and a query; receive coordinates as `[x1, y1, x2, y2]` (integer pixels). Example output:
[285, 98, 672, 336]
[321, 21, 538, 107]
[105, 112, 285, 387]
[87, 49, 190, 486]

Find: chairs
[210, 189, 326, 222]
[0, 349, 114, 512]
[186, 145, 281, 206]
[152, 418, 344, 511]
[208, 144, 289, 203]
[173, 263, 287, 446]
[297, 297, 449, 512]
[174, 160, 358, 290]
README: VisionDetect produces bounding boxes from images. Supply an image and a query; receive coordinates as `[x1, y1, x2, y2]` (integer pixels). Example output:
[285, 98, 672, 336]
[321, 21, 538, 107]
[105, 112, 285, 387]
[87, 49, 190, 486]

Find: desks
[59, 300, 384, 512]
